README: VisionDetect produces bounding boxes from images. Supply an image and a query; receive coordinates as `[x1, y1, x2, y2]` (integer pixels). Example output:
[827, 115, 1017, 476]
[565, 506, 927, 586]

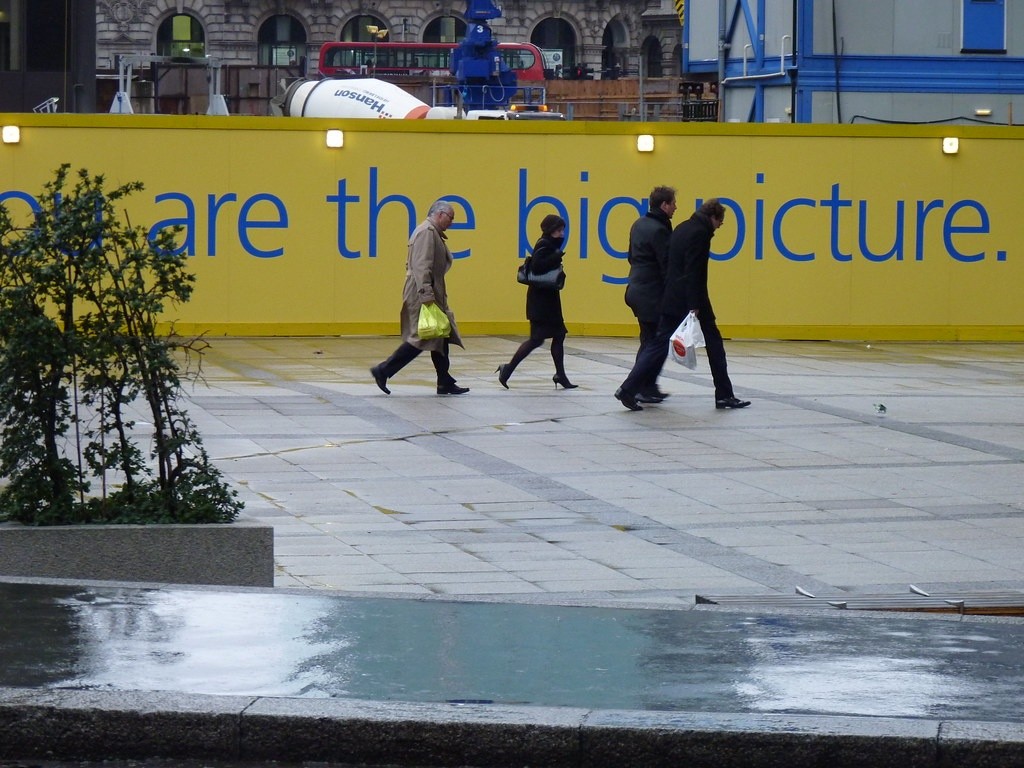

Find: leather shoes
[371, 365, 391, 394]
[716, 395, 751, 408]
[648, 387, 668, 398]
[635, 392, 664, 403]
[437, 383, 469, 394]
[614, 387, 643, 411]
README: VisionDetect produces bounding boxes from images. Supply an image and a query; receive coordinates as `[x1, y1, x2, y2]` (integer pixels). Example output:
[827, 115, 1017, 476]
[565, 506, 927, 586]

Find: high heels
[495, 363, 509, 389]
[553, 373, 579, 388]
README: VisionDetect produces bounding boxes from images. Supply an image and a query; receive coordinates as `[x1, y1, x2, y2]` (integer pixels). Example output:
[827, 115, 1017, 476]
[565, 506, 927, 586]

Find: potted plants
[0, 162, 275, 589]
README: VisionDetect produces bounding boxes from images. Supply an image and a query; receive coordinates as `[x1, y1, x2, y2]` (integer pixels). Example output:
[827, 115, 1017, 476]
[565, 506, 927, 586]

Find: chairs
[423, 64, 431, 68]
[518, 60, 524, 68]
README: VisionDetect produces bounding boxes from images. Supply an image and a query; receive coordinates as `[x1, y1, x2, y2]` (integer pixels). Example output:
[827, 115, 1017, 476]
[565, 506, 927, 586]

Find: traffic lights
[578, 63, 594, 80]
[563, 63, 583, 80]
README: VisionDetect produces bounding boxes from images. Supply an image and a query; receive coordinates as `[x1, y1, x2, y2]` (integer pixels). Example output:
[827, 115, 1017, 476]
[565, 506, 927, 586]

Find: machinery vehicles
[270, 76, 564, 121]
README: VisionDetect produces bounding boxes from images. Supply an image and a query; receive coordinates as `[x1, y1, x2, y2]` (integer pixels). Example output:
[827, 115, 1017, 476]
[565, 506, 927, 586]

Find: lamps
[326, 129, 343, 148]
[942, 136, 959, 153]
[637, 134, 656, 150]
[3, 125, 20, 144]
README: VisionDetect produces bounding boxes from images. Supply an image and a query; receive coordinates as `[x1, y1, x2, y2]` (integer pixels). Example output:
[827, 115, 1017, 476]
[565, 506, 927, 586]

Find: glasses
[443, 212, 453, 221]
[717, 219, 723, 226]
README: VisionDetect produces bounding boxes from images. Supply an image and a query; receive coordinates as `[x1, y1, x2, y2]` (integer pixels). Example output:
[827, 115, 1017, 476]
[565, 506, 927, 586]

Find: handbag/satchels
[418, 302, 451, 339]
[669, 312, 697, 370]
[517, 247, 566, 290]
[689, 311, 706, 348]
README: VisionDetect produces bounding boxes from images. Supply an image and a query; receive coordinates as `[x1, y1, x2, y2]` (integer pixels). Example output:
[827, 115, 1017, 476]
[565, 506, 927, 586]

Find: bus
[318, 42, 554, 81]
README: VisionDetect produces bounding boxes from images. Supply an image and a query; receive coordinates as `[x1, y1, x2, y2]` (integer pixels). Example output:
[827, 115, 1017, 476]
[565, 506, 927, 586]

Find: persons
[366, 60, 372, 67]
[614, 201, 751, 411]
[370, 202, 469, 394]
[624, 187, 677, 404]
[410, 58, 418, 67]
[495, 215, 579, 389]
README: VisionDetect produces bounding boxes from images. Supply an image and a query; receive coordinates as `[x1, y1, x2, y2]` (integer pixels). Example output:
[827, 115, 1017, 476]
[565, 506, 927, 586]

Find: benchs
[695, 584, 1024, 617]
